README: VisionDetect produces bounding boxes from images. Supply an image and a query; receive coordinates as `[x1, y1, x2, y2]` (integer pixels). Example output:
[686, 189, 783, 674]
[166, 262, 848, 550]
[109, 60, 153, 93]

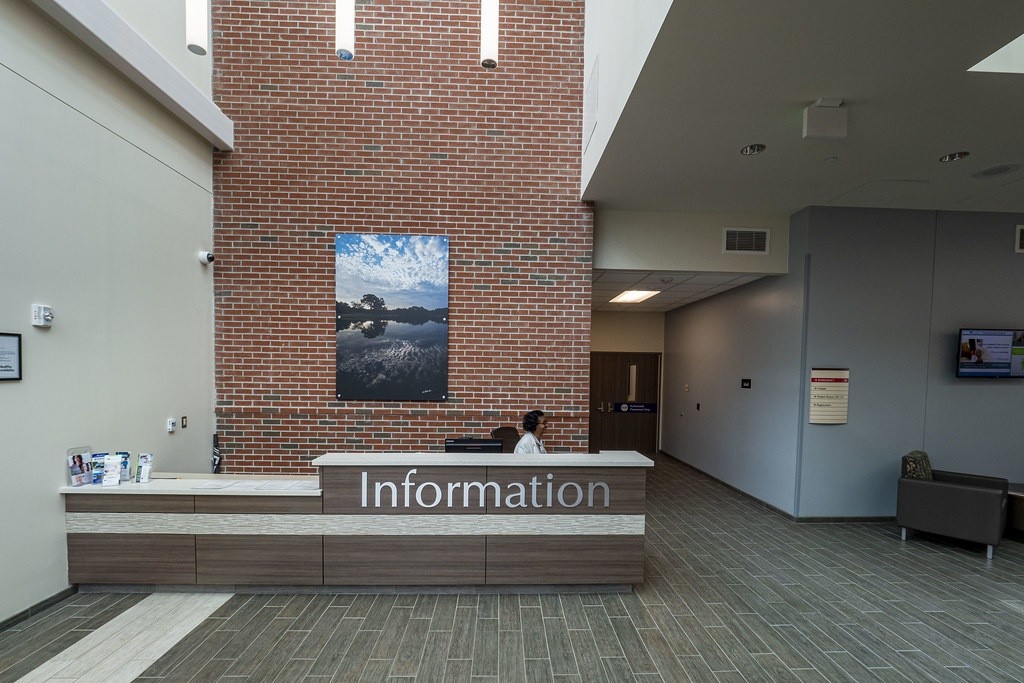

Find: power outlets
[168, 419, 177, 432]
[181, 416, 188, 429]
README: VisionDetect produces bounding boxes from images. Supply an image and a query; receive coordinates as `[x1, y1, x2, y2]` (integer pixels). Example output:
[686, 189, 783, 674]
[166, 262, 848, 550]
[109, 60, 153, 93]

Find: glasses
[537, 420, 548, 425]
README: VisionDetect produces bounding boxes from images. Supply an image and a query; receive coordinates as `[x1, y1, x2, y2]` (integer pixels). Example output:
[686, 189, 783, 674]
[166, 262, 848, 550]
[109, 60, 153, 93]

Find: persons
[513, 410, 549, 455]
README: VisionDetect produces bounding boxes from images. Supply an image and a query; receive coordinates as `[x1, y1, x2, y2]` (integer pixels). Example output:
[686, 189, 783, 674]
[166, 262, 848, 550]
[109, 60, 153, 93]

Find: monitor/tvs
[445, 438, 503, 454]
[955, 328, 1024, 379]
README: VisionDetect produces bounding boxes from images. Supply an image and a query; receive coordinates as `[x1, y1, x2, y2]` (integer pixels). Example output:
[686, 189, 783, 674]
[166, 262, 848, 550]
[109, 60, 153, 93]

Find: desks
[59, 447, 654, 590]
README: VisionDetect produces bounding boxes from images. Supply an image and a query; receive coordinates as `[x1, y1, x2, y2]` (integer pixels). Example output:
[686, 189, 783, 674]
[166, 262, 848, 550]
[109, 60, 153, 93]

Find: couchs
[893, 448, 1013, 563]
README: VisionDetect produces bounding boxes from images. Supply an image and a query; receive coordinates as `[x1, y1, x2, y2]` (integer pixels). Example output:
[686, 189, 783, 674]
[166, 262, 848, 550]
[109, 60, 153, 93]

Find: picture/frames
[0, 332, 23, 382]
